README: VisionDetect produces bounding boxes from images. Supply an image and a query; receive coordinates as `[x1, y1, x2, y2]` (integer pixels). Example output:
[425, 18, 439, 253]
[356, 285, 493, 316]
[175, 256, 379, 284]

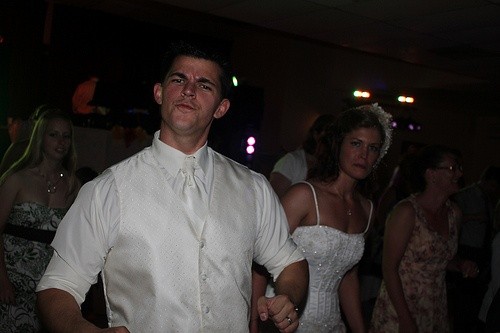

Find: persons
[0, 110, 82, 333]
[36, 48, 310, 333]
[0, 45, 500, 333]
[372, 148, 479, 333]
[250, 105, 390, 333]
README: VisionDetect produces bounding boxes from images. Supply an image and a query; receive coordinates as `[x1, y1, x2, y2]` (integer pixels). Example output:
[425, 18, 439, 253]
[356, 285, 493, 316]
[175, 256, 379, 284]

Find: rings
[285, 316, 292, 325]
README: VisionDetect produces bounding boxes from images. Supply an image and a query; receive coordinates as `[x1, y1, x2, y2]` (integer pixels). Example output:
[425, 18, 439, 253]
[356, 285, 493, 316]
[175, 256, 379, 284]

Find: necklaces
[37, 165, 64, 193]
[329, 181, 356, 216]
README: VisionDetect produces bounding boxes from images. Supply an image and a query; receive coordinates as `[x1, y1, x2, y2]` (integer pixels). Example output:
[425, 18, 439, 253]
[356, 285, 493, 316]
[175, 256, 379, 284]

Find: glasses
[435, 165, 463, 175]
